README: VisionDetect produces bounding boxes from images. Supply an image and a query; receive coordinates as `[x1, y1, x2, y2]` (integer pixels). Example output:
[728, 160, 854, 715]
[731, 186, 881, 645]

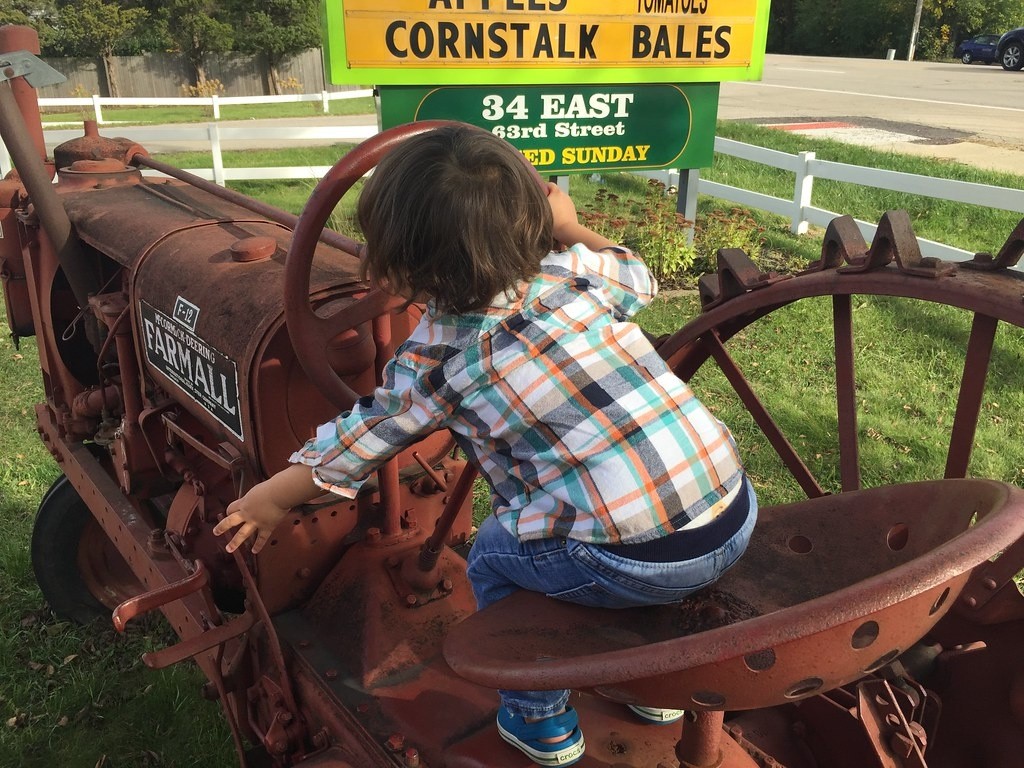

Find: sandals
[497, 700, 586, 768]
[627, 701, 684, 726]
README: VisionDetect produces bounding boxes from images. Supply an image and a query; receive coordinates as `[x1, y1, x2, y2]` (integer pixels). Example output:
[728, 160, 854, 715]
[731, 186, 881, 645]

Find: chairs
[442, 477, 1024, 768]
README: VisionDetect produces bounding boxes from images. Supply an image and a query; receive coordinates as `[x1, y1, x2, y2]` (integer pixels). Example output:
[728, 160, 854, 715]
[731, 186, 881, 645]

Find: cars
[954, 34, 1001, 65]
[994, 30, 1023, 71]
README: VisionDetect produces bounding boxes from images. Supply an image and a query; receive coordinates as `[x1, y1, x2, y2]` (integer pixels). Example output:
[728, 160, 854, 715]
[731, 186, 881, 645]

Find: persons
[212, 122, 758, 768]
